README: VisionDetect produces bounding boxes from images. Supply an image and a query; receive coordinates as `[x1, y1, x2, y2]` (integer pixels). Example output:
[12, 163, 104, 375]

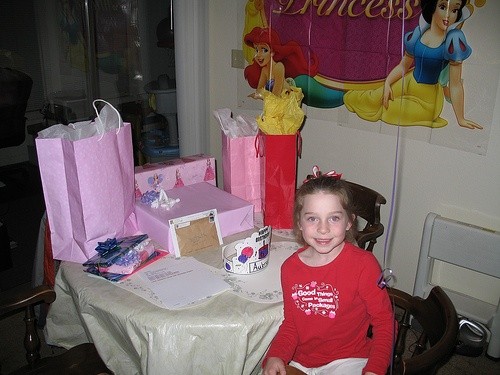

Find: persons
[261, 165, 398, 375]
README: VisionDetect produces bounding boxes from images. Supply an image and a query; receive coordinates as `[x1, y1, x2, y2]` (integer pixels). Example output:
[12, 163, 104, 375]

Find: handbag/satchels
[221, 126, 262, 213]
[255, 127, 302, 229]
[36, 99, 141, 263]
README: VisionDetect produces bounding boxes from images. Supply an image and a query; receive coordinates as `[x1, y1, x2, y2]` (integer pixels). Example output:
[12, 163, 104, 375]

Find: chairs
[0, 285, 108, 375]
[386, 286, 459, 375]
[307, 175, 386, 252]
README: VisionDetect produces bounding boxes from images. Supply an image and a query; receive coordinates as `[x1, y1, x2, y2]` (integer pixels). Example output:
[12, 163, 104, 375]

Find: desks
[44, 228, 304, 375]
